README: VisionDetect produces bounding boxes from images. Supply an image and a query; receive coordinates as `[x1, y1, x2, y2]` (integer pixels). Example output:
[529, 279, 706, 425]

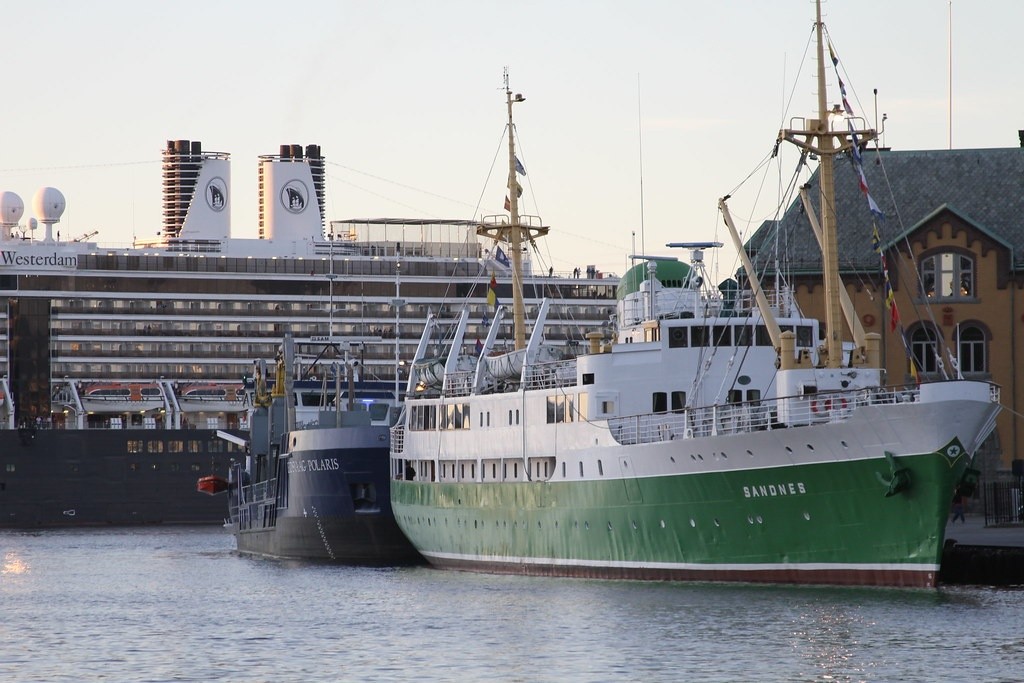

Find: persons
[586, 267, 595, 279]
[47, 415, 52, 428]
[35, 415, 42, 429]
[573, 266, 581, 280]
[548, 266, 554, 277]
[952, 482, 967, 526]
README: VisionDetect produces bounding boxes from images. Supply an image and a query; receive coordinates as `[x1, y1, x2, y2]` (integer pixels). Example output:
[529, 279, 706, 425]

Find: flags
[829, 42, 920, 385]
[474, 155, 526, 353]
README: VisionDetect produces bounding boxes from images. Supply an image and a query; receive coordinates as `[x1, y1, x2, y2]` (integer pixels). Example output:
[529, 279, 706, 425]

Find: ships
[1, 138, 620, 528]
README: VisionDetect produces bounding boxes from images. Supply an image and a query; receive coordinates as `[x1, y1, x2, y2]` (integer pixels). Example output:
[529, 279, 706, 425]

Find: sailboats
[386, 0, 1004, 591]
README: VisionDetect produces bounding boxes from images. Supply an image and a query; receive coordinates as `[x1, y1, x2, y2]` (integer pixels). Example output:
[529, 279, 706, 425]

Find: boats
[196, 223, 422, 569]
